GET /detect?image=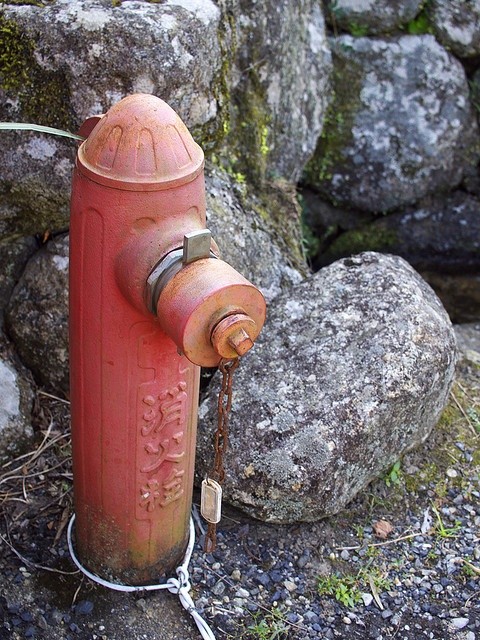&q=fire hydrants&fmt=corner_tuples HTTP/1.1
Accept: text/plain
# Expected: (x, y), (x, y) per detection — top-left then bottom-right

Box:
(69, 92), (267, 588)
